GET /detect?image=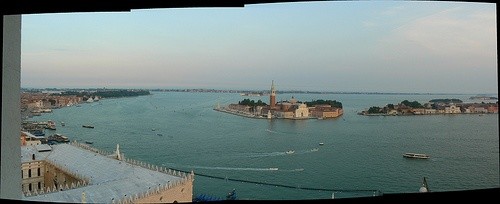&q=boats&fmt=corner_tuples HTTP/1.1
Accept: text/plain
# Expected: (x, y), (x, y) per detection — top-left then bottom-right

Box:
(82, 124), (94, 128)
(31, 108), (53, 116)
(49, 133), (70, 144)
(270, 167), (278, 171)
(402, 153), (431, 159)
(319, 142), (324, 146)
(44, 120), (58, 129)
(285, 150), (295, 154)
